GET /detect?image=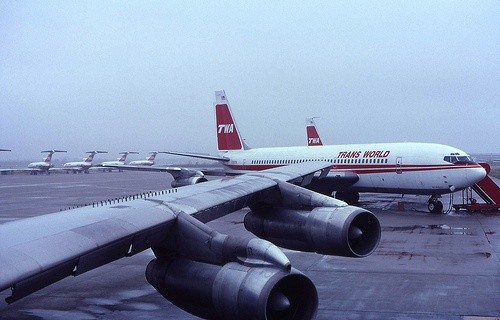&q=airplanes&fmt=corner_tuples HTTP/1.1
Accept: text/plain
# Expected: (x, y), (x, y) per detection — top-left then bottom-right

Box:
(1, 166), (381, 320)
(0, 149), (167, 176)
(159, 90), (488, 214)
